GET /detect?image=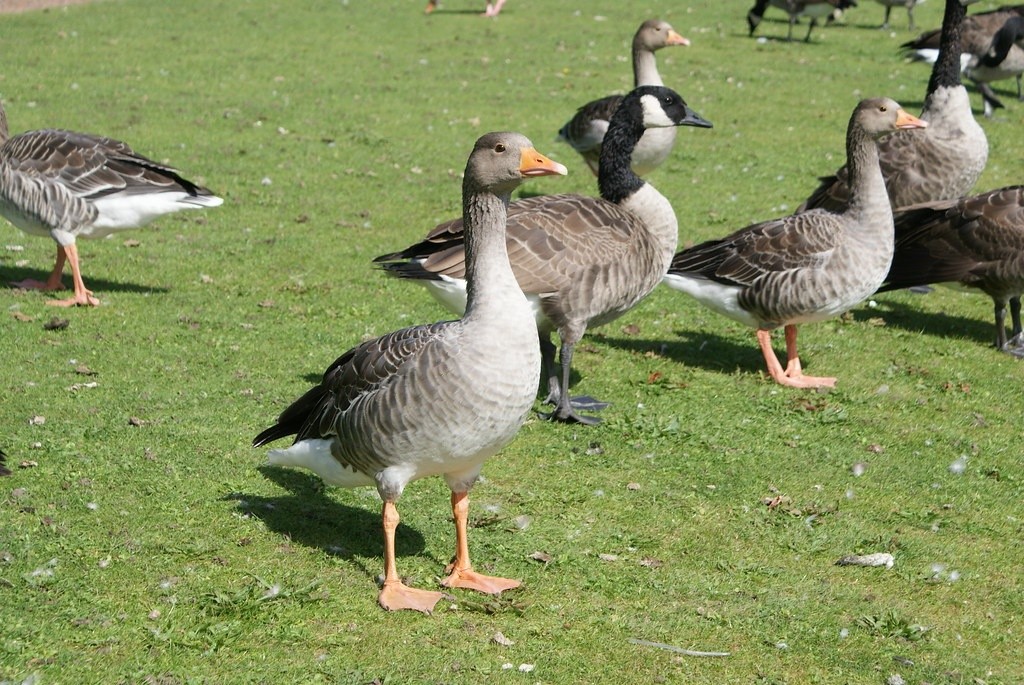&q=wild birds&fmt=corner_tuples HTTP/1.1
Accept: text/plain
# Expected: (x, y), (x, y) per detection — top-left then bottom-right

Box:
(0, 102), (226, 308)
(249, 0), (1024, 614)
